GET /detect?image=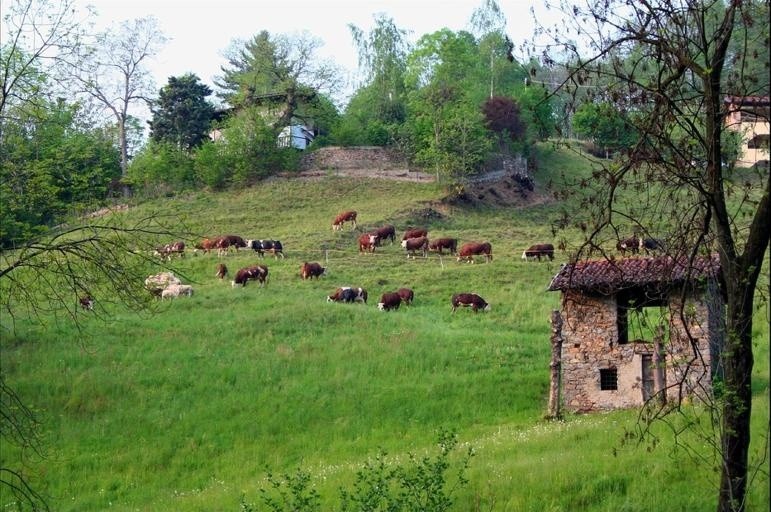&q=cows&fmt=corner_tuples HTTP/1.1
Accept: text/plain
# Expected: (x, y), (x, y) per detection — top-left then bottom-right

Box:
(332, 210), (357, 232)
(154, 241), (184, 264)
(522, 244), (554, 262)
(451, 293), (488, 314)
(144, 272), (193, 303)
(215, 264), (268, 290)
(80, 296), (95, 312)
(357, 224), (493, 265)
(377, 288), (414, 312)
(195, 234), (284, 261)
(616, 238), (667, 256)
(327, 287), (367, 304)
(301, 263), (325, 281)
